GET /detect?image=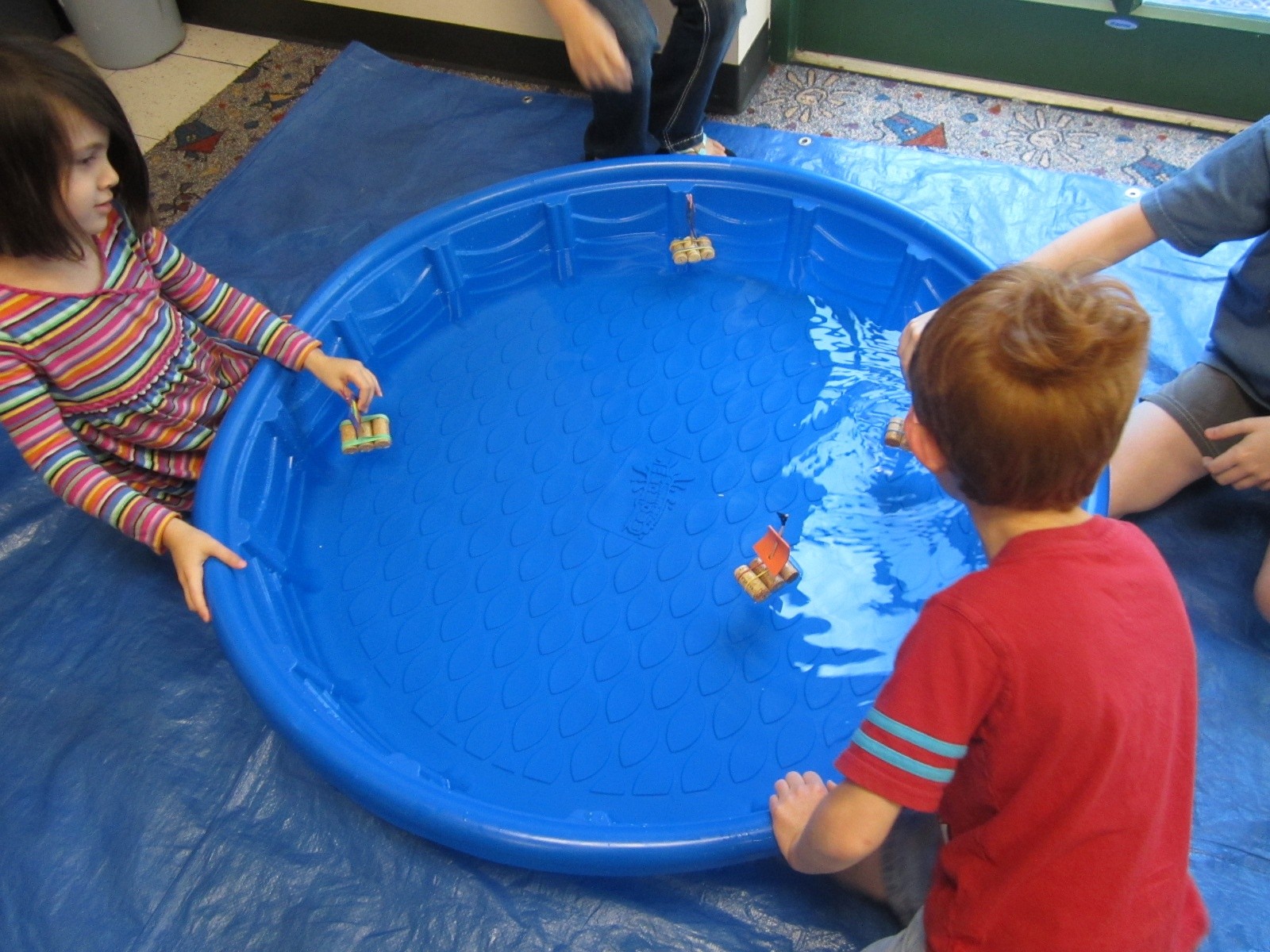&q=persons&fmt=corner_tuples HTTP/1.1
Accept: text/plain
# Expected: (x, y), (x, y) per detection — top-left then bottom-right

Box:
(899, 113), (1270, 624)
(0, 35), (385, 624)
(540, 0), (746, 160)
(767, 260), (1208, 951)
